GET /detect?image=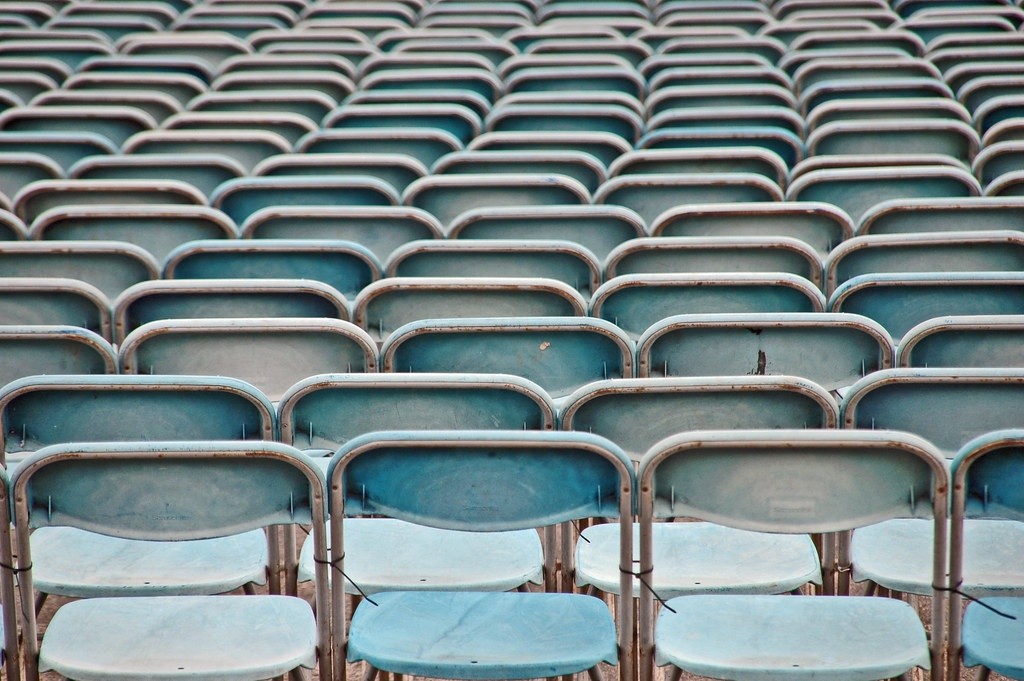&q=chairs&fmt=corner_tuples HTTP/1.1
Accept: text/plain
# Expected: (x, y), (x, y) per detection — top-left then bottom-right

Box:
(0, 0), (1024, 681)
